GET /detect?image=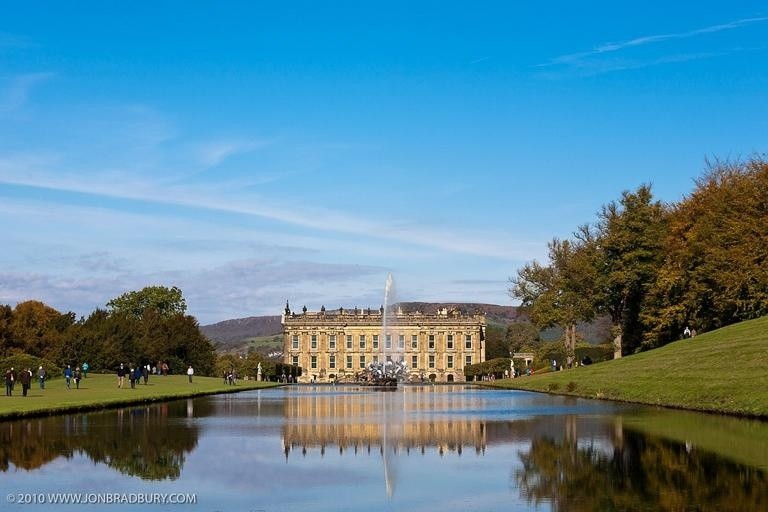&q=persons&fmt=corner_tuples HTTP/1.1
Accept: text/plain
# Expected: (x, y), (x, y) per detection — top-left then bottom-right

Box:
(4, 366), (45, 397)
(64, 362), (88, 389)
(550, 357), (557, 372)
(223, 368), (239, 385)
(488, 365), (535, 382)
(117, 360), (169, 389)
(281, 372), (293, 383)
(566, 352), (592, 368)
(684, 327), (697, 339)
(187, 365), (194, 383)
